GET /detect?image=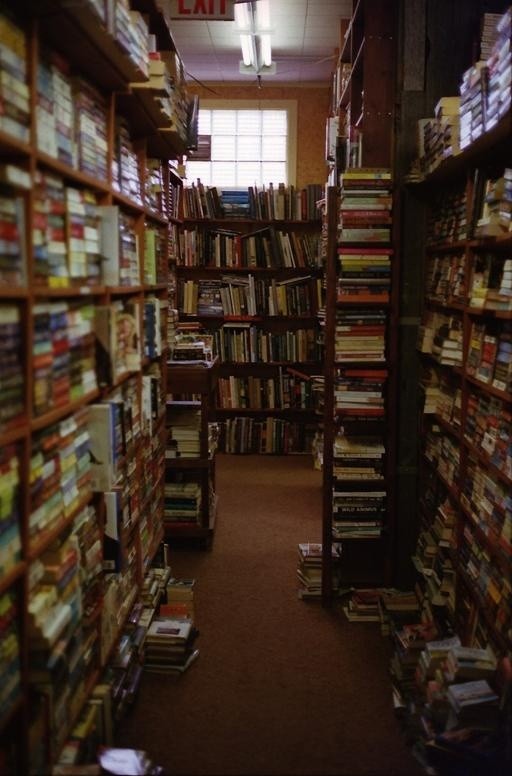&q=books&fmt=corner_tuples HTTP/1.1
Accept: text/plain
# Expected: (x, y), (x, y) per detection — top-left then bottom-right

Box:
(1, 1), (221, 775)
(221, 182), (297, 455)
(296, 1), (511, 775)
